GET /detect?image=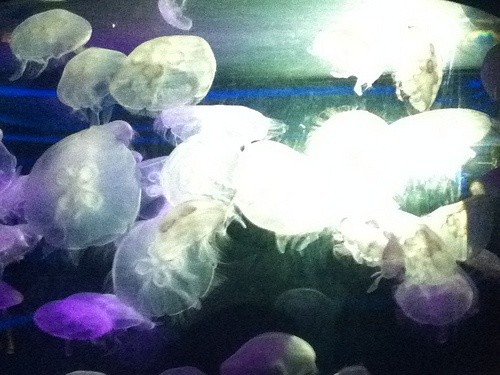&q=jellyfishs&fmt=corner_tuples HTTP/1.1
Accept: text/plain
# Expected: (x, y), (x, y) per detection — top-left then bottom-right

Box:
(1, 0), (499, 375)
